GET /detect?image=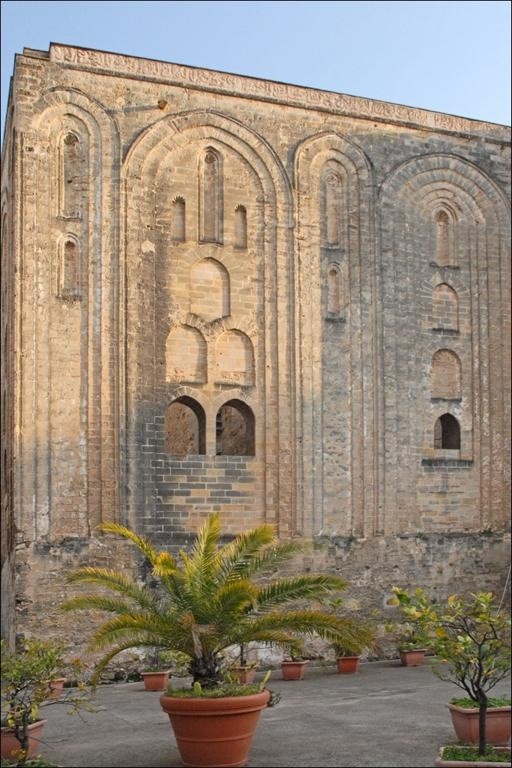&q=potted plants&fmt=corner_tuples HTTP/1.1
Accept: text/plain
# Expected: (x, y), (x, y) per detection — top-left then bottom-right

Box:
(0, 638), (106, 768)
(383, 584), (511, 767)
(62, 510), (377, 768)
